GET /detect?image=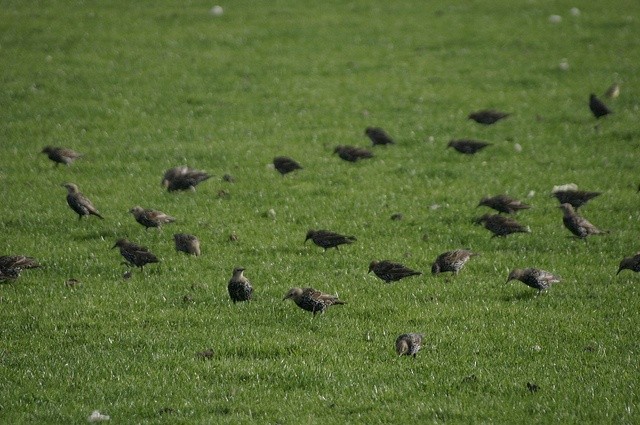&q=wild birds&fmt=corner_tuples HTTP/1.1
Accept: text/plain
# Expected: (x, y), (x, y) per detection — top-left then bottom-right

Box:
(173, 232), (201, 257)
(229, 264), (255, 305)
(396, 333), (422, 358)
(445, 139), (494, 155)
(474, 194), (533, 216)
(303, 229), (358, 253)
(432, 248), (479, 277)
(128, 206), (178, 233)
(273, 156), (304, 177)
(601, 82), (620, 97)
(332, 144), (376, 163)
(504, 267), (562, 298)
(160, 164), (216, 194)
(554, 203), (610, 239)
(615, 253), (640, 275)
(466, 108), (514, 125)
(367, 258), (423, 284)
(60, 183), (105, 221)
(281, 287), (347, 319)
(0, 255), (40, 284)
(364, 126), (396, 148)
(551, 190), (604, 213)
(471, 214), (532, 241)
(589, 93), (617, 121)
(38, 145), (84, 170)
(110, 238), (160, 272)
